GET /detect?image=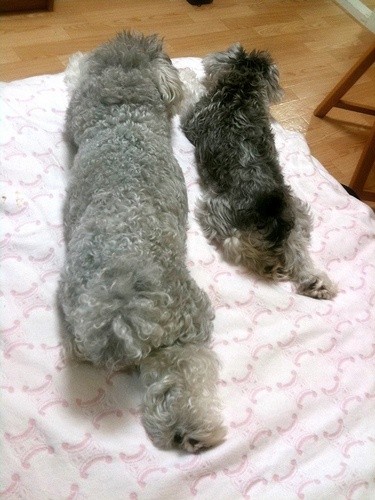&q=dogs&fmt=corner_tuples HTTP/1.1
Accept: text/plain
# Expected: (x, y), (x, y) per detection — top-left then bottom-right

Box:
(56, 29), (227, 456)
(180, 44), (339, 299)
